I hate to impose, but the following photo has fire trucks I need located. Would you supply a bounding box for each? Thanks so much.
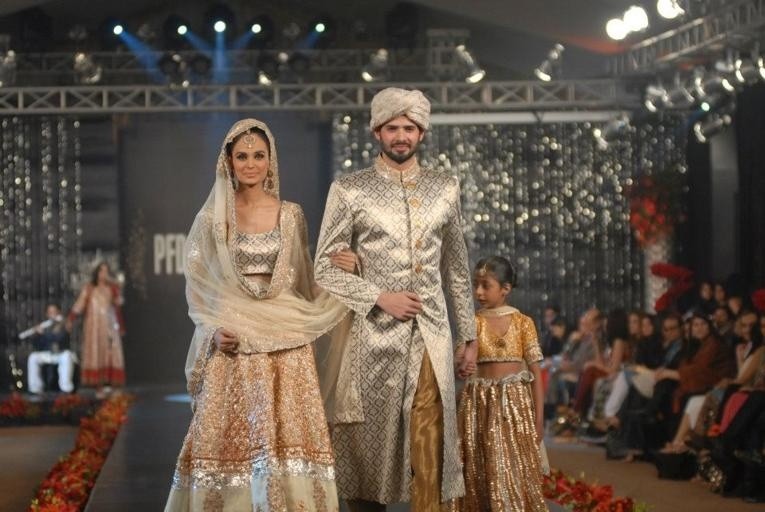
[18,315,62,339]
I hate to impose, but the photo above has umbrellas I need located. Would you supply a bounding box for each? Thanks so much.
[661,435,690,454]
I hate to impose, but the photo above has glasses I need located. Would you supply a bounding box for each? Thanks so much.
[534,42,567,82]
[455,43,486,87]
[694,110,733,144]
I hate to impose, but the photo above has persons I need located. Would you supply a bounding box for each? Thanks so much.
[67,261,127,390]
[544,278,765,469]
[456,257,551,512]
[314,88,479,512]
[24,302,75,395]
[165,119,356,512]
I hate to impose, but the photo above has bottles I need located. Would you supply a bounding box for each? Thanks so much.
[369,87,431,130]
